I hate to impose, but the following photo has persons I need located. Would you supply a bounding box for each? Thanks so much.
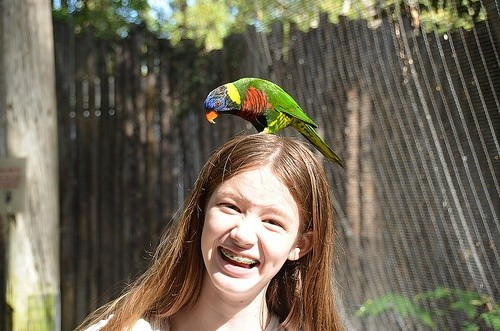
[74,132,345,331]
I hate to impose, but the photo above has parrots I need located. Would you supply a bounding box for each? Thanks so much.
[203,78,346,169]
